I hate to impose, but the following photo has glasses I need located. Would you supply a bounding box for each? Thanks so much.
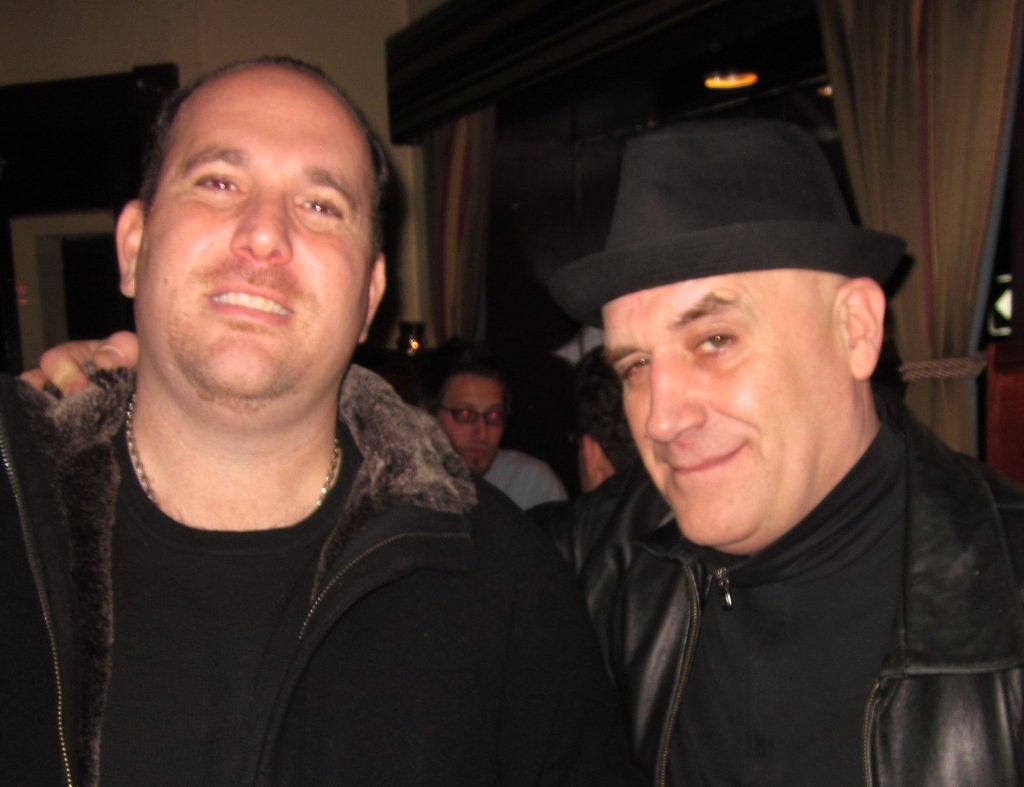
[438,403,506,426]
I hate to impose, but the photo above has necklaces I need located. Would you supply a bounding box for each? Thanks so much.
[125,386,340,523]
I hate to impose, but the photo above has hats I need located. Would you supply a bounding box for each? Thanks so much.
[548,120,912,330]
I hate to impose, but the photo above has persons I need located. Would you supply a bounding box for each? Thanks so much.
[570,343,639,495]
[0,54,642,787]
[416,334,570,511]
[18,116,1024,787]
